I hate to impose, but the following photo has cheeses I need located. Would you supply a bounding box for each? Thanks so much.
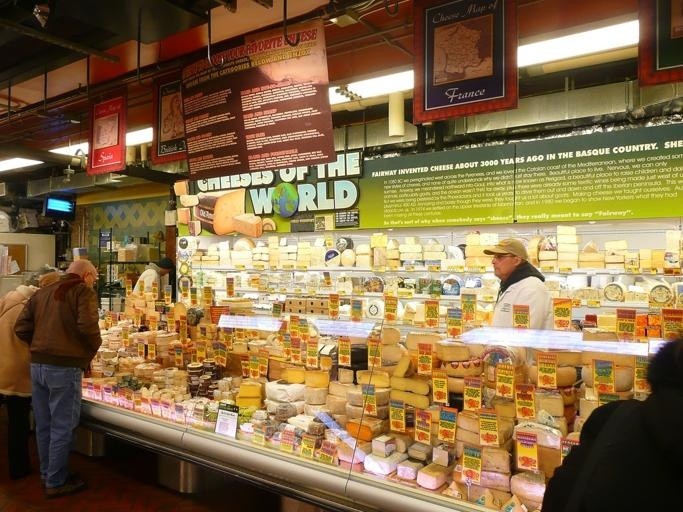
[81,224,683,512]
[173,180,276,237]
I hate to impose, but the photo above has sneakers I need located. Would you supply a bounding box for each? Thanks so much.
[44,478,87,497]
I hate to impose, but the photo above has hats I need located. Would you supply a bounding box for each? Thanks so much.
[484,239,528,261]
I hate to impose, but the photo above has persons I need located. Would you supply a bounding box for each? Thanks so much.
[14,258,103,499]
[0,270,62,481]
[483,239,554,329]
[133,258,174,300]
[540,331,683,512]
[164,95,184,138]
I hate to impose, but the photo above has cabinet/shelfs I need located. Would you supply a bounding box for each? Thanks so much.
[189,237,681,313]
[80,296,682,510]
